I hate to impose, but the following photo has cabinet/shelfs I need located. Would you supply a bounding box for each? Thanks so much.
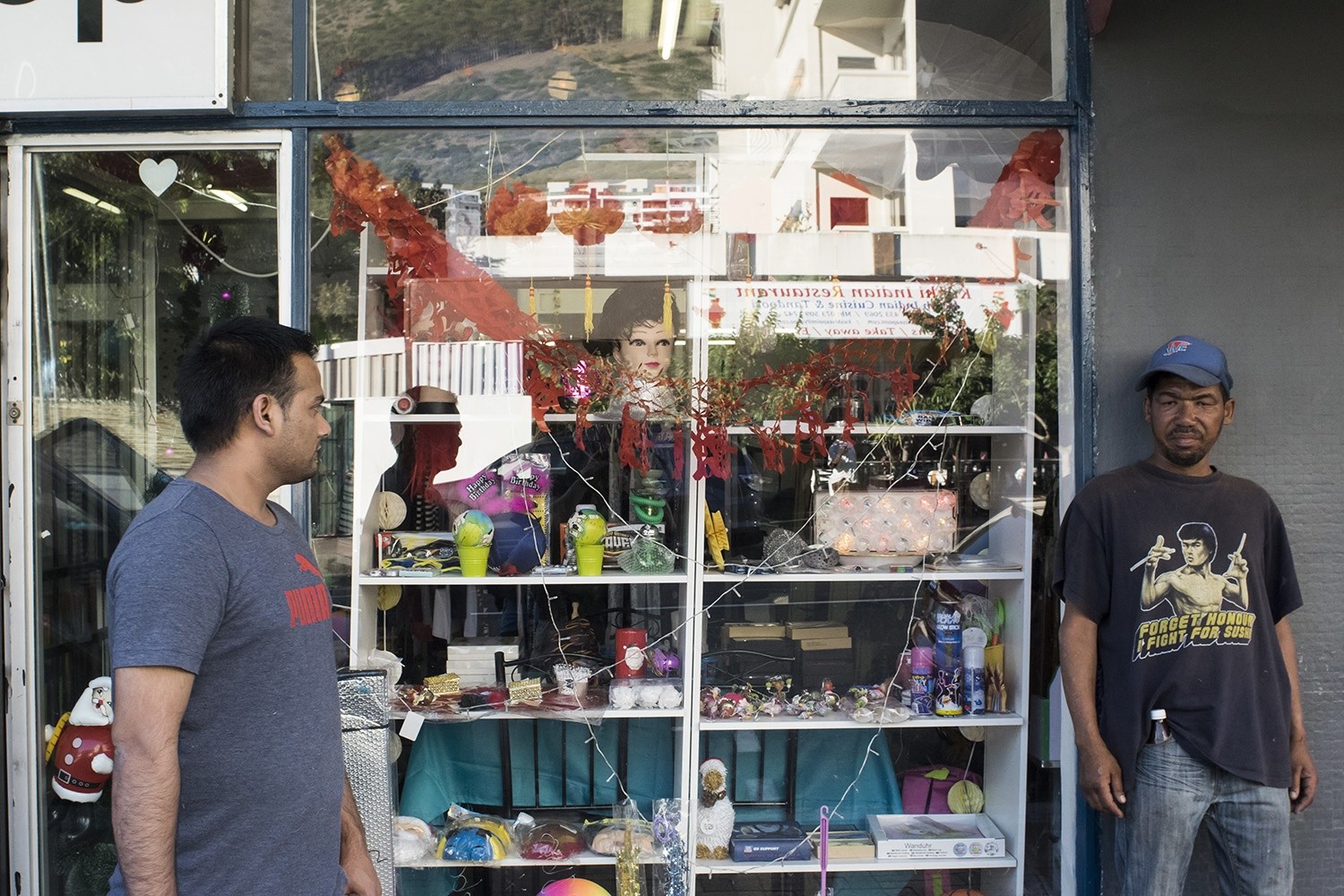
[698,272,1041,894]
[342,182,701,896]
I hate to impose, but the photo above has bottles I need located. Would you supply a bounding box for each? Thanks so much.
[1149,708,1169,745]
[965,645,986,714]
[910,647,933,714]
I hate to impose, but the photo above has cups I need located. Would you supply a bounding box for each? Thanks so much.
[574,545,604,577]
[614,626,648,678]
[456,548,489,577]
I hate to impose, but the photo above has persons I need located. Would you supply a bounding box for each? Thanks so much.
[586,282,682,418]
[1048,332,1320,895]
[827,430,860,487]
[103,316,383,896]
[367,384,467,691]
[45,676,113,849]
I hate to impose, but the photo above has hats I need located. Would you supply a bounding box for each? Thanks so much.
[1136,335,1233,398]
